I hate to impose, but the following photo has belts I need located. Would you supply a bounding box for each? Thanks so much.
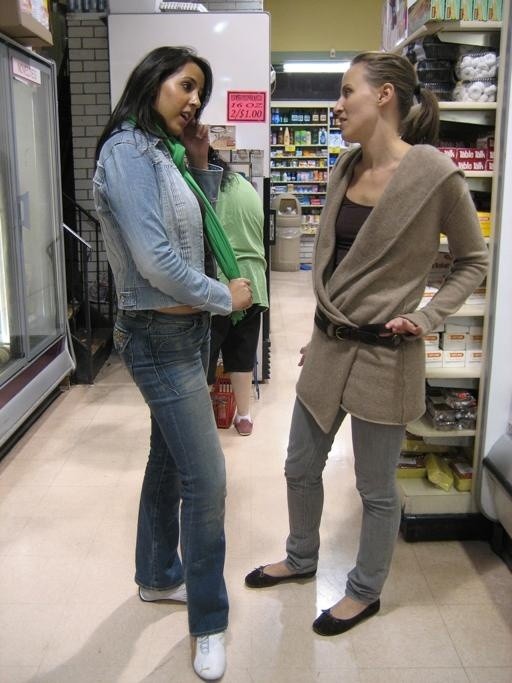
[314,305,404,348]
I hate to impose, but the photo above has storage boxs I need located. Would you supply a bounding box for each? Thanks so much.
[0,0,54,48]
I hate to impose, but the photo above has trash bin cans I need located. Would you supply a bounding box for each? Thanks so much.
[271,193,303,272]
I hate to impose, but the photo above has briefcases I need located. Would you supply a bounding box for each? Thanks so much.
[210,375,237,428]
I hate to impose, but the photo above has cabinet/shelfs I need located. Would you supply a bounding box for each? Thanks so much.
[330,106,361,177]
[382,21,504,545]
[268,106,329,253]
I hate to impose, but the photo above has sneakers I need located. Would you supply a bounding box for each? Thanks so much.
[193,630,226,680]
[234,414,253,435]
[139,580,188,602]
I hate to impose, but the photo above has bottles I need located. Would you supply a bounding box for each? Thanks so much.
[270,125,328,144]
[270,107,325,123]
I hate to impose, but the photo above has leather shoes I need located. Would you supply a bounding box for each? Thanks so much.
[245,563,316,587]
[313,597,380,635]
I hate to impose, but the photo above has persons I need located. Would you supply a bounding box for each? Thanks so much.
[207,146,270,437]
[92,45,255,683]
[247,52,490,634]
